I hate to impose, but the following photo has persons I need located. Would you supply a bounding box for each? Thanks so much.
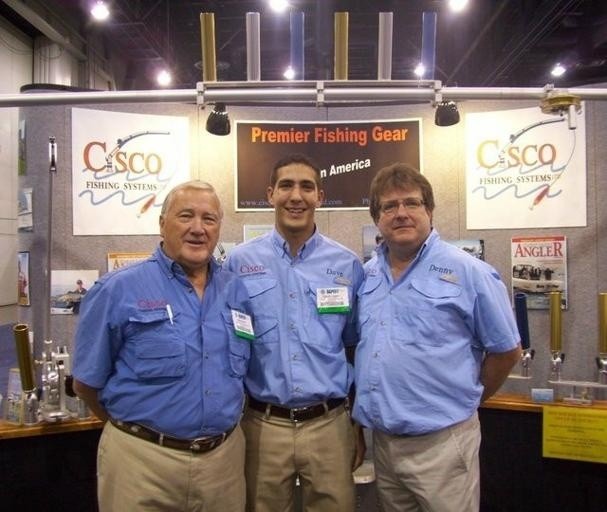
[73,280,87,295]
[351,162,523,511]
[228,155,368,510]
[71,180,252,511]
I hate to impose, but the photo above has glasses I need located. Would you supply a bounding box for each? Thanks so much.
[381,198,423,212]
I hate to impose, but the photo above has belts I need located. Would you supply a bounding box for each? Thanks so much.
[106,413,238,453]
[398,433,429,438]
[249,398,344,420]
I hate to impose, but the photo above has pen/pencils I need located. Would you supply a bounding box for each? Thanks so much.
[166,304,175,326]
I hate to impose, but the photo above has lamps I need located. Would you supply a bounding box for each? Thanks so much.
[197,78,232,136]
[431,80,462,126]
